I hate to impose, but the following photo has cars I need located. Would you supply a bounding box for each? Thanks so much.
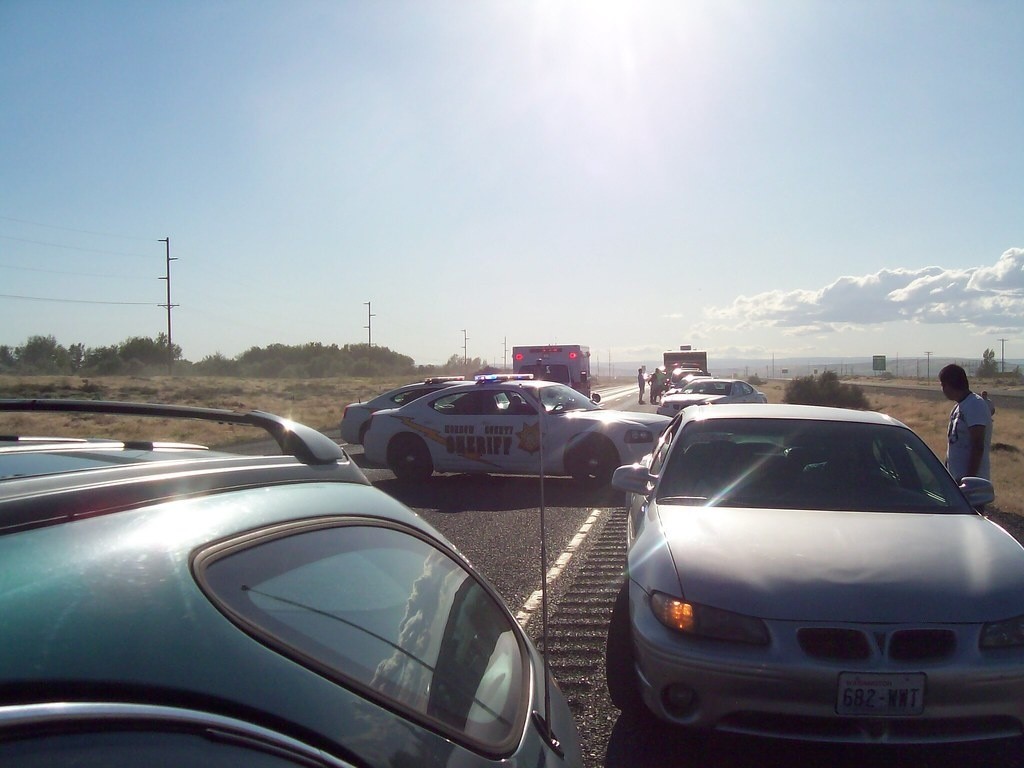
[661,376,722,400]
[361,377,671,490]
[658,379,768,416]
[343,377,566,447]
[605,404,1024,749]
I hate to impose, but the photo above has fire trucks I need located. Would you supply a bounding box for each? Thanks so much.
[512,345,591,397]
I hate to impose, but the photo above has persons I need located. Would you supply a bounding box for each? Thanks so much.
[638,368,646,404]
[647,368,670,404]
[507,396,529,411]
[938,364,995,514]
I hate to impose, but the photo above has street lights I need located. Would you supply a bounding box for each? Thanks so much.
[997,339,1008,372]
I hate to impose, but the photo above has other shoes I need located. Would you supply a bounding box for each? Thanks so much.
[639,400,645,405]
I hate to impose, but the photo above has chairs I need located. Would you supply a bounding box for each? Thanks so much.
[683,441,903,505]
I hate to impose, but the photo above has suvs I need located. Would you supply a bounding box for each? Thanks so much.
[666,366,705,390]
[1,396,579,768]
[663,345,711,383]
